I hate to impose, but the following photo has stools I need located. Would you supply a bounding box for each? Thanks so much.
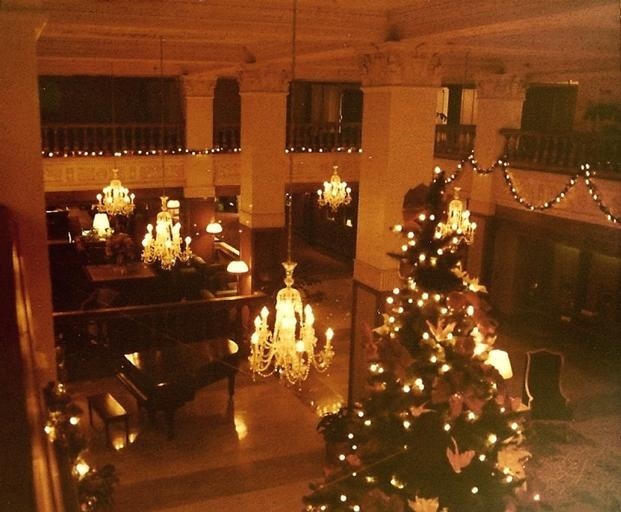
[87,391,129,447]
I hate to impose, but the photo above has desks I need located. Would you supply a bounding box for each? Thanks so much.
[86,261,226,305]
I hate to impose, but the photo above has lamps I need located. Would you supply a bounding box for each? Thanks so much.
[227,260,250,276]
[240,1,336,386]
[140,36,193,272]
[204,222,223,234]
[316,44,353,212]
[95,62,136,218]
[434,50,477,251]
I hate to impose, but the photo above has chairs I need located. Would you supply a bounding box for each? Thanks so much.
[522,350,571,445]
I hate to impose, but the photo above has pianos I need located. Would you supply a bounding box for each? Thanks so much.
[114,336,239,439]
